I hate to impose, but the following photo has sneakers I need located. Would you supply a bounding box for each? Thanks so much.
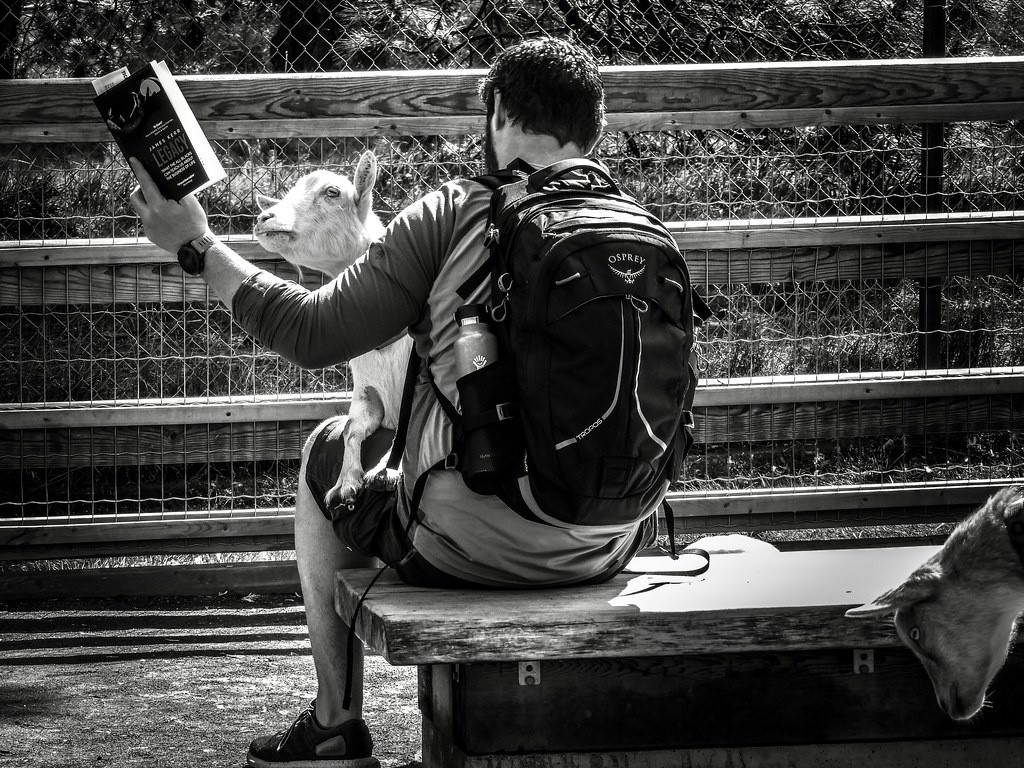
[246,698,380,768]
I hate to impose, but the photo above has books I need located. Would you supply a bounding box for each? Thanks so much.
[94,60,228,204]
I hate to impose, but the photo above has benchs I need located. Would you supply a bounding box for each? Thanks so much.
[332,545,945,768]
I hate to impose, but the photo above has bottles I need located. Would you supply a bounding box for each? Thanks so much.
[452,303,499,379]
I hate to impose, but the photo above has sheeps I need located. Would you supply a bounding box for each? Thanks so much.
[251,150,416,508]
[844,483,1024,722]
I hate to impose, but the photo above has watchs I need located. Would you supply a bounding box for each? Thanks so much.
[177,231,220,276]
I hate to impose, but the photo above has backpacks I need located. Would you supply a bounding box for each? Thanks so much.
[419,157,702,528]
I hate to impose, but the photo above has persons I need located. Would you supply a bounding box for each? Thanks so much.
[127,38,660,768]
